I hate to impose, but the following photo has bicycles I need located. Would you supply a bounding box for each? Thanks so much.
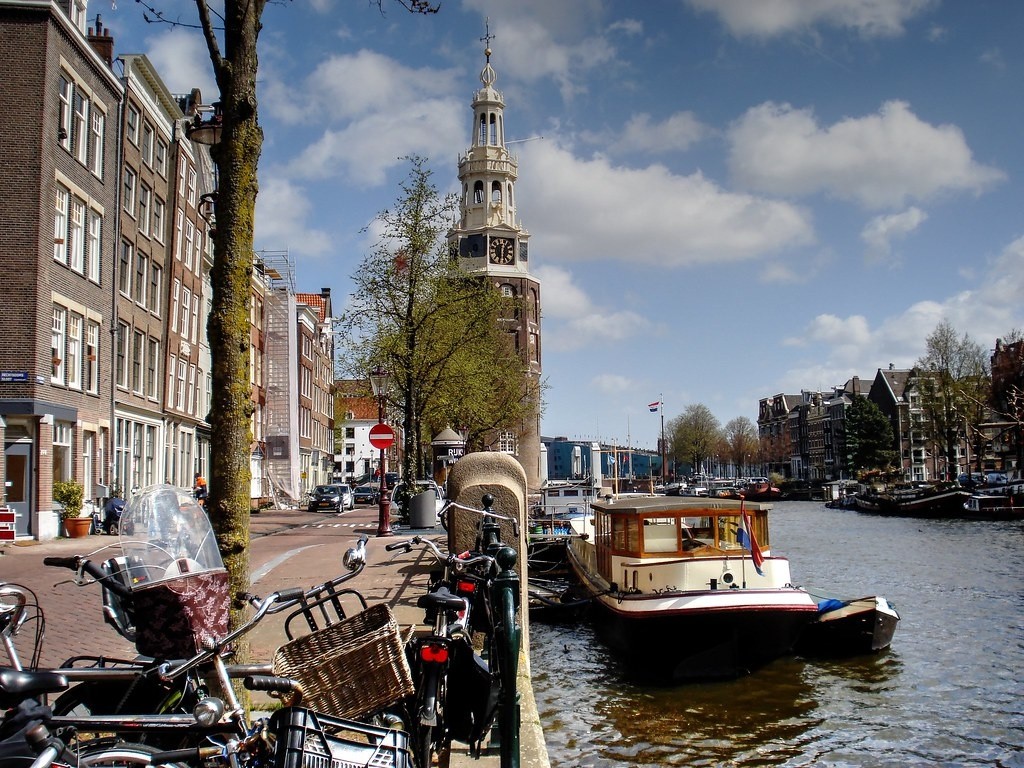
[0,484,520,768]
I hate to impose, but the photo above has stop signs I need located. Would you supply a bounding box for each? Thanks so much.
[369,424,394,449]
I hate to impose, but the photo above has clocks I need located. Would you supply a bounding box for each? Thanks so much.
[490,238,514,265]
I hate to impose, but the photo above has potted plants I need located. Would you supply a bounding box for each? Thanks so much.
[53,479,93,539]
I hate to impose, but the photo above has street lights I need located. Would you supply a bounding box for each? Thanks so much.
[370,450,374,469]
[368,362,396,537]
[349,449,354,484]
[743,453,751,473]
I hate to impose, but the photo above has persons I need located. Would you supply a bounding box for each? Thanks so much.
[193,473,207,509]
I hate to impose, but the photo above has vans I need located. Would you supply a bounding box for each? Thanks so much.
[747,477,775,486]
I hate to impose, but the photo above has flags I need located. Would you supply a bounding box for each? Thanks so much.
[623,455,628,462]
[736,502,766,577]
[608,455,616,467]
[648,401,660,413]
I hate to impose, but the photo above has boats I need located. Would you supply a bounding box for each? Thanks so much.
[565,496,818,646]
[653,480,745,499]
[739,482,783,500]
[963,495,1024,519]
[855,487,972,519]
[792,596,900,654]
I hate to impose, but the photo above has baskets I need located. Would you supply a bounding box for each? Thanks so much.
[271,603,415,726]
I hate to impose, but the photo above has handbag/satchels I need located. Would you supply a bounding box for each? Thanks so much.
[448,637,502,744]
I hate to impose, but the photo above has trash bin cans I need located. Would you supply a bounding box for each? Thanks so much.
[408,489,436,527]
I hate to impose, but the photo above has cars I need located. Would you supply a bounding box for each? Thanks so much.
[987,475,1008,484]
[353,486,380,506]
[1005,479,1024,491]
[331,484,355,511]
[387,480,446,529]
[958,475,981,485]
[351,474,376,490]
[373,477,381,483]
[308,485,344,513]
[386,472,400,485]
